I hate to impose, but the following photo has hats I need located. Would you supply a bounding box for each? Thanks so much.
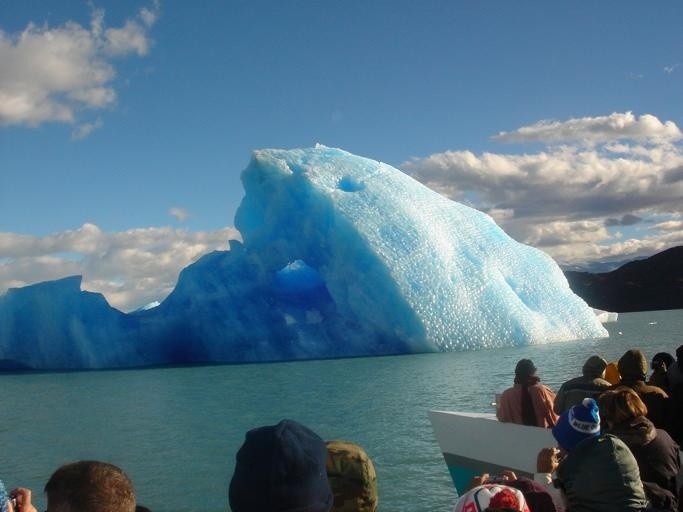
[453,478,557,512]
[552,397,601,453]
[229,419,378,512]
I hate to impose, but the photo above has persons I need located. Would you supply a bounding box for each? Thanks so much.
[228,418,335,511]
[4,459,136,512]
[454,345,681,510]
[325,440,378,511]
